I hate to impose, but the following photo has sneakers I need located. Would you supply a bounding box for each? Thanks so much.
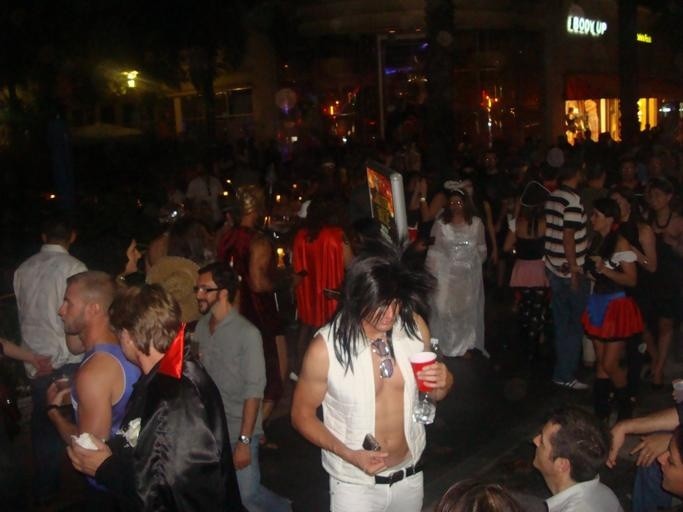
[549,359,595,390]
[288,367,300,382]
[640,362,667,394]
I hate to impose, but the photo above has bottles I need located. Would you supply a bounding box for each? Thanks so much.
[411,336,442,426]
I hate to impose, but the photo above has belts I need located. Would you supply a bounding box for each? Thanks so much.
[375,464,423,485]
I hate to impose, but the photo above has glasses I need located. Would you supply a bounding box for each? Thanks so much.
[371,338,393,379]
[192,286,221,294]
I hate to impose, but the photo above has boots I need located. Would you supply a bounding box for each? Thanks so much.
[594,376,637,424]
